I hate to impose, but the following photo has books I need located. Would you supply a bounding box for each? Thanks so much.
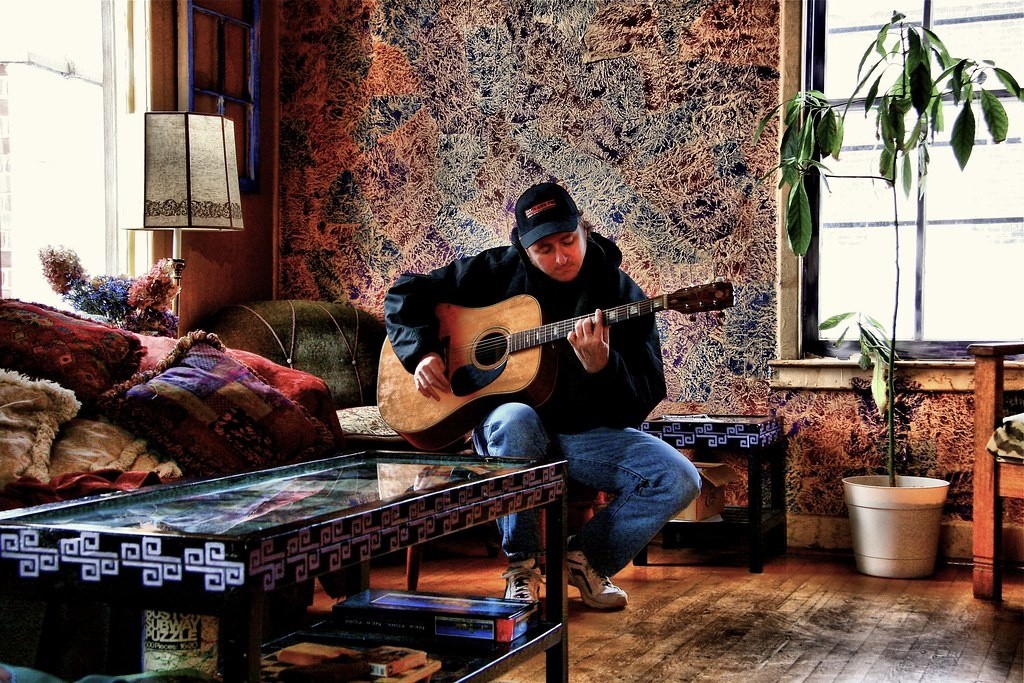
[279,642,442,683]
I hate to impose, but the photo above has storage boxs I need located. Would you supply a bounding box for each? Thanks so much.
[331,586,544,645]
[671,461,727,521]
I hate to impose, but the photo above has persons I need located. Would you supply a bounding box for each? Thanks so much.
[383,182,702,609]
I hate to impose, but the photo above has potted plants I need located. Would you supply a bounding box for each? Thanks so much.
[748,9,1024,581]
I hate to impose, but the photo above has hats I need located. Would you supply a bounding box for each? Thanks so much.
[515,182,579,249]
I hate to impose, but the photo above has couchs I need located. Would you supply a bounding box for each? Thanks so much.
[0,301,346,620]
[198,298,385,407]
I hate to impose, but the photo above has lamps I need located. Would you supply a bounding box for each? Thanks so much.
[115,107,246,326]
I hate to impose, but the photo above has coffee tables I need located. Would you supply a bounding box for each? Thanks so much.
[0,443,574,683]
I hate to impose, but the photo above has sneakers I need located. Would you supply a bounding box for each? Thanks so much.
[501,559,546,601]
[567,535,628,609]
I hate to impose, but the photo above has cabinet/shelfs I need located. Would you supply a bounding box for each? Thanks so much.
[633,411,786,573]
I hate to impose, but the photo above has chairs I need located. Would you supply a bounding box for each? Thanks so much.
[965,340,1024,615]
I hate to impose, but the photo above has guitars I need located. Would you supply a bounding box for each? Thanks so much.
[376,275,738,453]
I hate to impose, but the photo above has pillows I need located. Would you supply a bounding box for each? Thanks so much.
[0,296,148,409]
[0,363,82,486]
[93,330,341,483]
